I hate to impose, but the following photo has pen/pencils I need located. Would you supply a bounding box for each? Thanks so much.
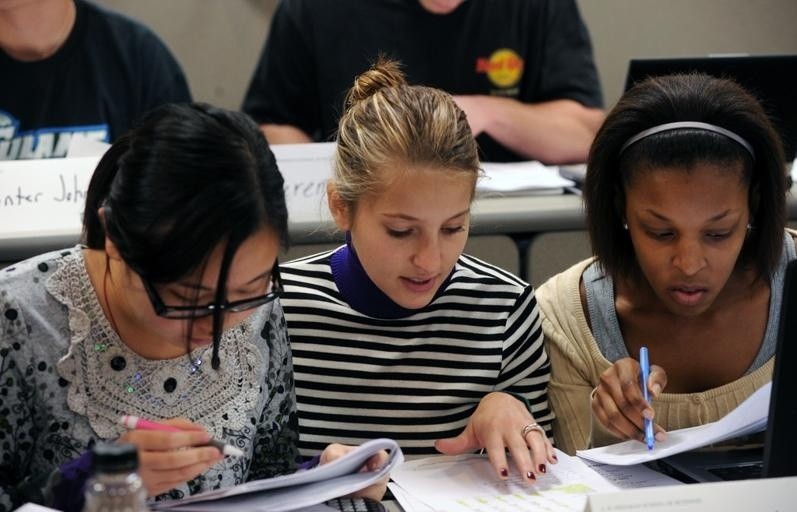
[120,413,251,461]
[639,346,654,452]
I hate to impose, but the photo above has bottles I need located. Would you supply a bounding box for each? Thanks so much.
[81,441,148,512]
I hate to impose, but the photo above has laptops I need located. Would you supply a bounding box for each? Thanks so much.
[559,55,796,186]
[644,261,797,485]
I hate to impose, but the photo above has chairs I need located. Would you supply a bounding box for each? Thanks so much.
[458,230,523,279]
[523,229,597,292]
[268,236,348,271]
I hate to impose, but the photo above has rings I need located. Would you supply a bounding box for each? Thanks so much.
[520,423,545,439]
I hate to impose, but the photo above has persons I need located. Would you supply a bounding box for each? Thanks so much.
[272,53,559,486]
[0,0,194,163]
[0,101,390,511]
[238,0,605,166]
[535,71,795,457]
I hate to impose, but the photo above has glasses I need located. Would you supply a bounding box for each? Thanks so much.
[140,255,284,320]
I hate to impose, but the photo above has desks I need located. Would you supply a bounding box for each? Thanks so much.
[0,152,591,245]
